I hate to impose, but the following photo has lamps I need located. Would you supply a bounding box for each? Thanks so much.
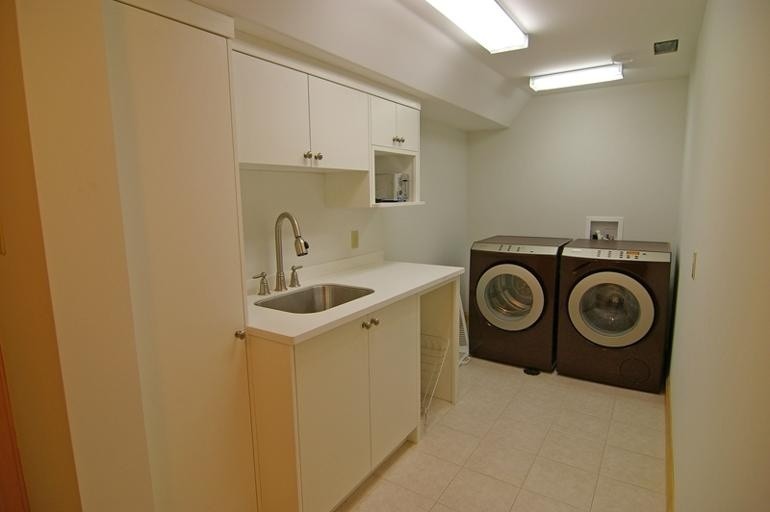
[528,62,624,93]
[427,0,529,55]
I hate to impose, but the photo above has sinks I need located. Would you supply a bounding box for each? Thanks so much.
[254,284,375,313]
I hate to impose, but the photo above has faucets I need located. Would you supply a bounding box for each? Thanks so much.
[274,211,308,292]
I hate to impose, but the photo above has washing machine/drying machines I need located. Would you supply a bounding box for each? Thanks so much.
[556,238,672,394]
[468,235,573,373]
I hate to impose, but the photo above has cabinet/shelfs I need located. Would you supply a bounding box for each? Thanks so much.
[230,41,368,171]
[0,1,263,510]
[247,296,421,511]
[369,90,425,210]
